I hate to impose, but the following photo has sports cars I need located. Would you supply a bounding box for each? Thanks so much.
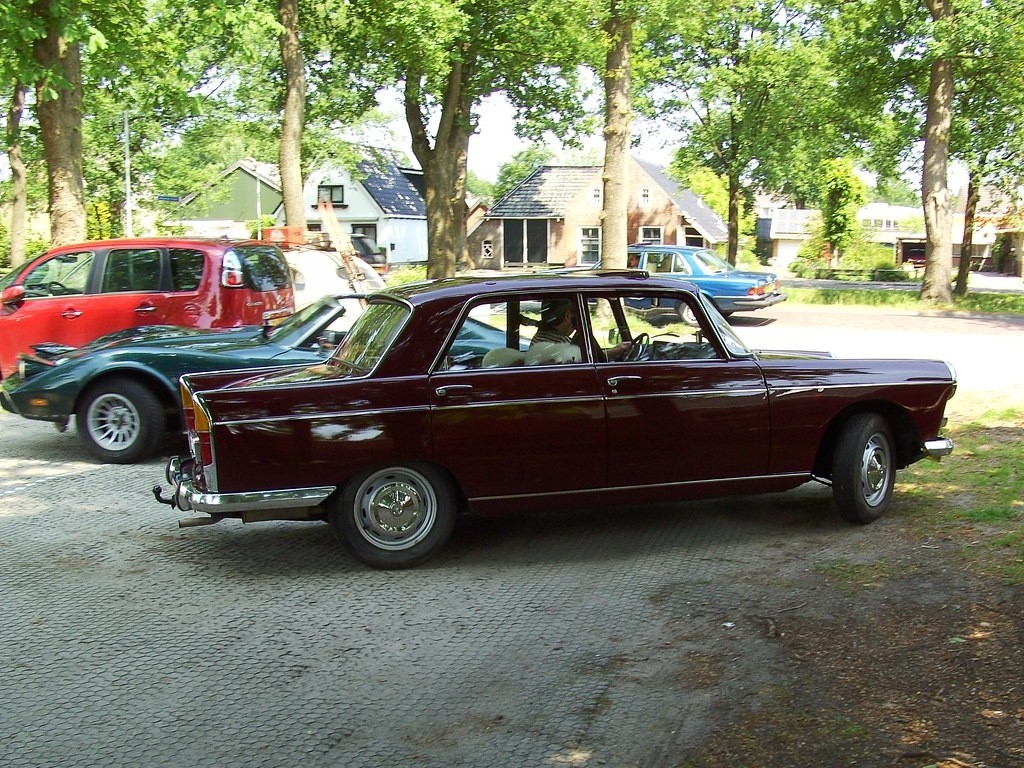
[1,293,534,464]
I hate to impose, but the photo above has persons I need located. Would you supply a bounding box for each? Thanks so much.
[529,296,631,362]
[629,254,638,268]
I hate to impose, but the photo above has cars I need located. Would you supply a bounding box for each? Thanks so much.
[279,247,391,335]
[0,236,295,384]
[153,263,958,571]
[590,241,788,322]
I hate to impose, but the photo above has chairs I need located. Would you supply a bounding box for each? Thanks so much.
[524,342,582,366]
[483,347,524,368]
